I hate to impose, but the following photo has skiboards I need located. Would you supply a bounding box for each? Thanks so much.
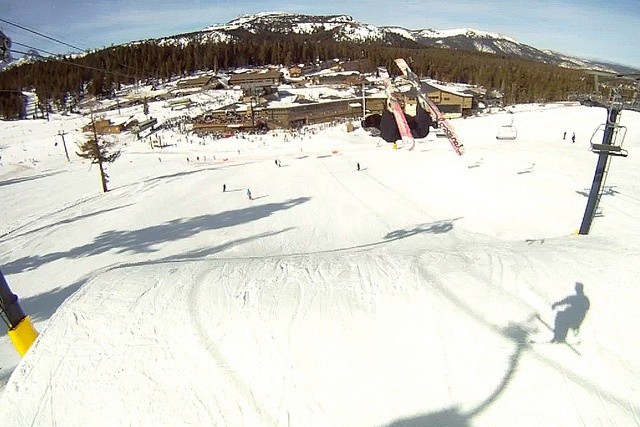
[377,59,465,156]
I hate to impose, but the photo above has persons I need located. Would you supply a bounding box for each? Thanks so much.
[361,90,438,143]
